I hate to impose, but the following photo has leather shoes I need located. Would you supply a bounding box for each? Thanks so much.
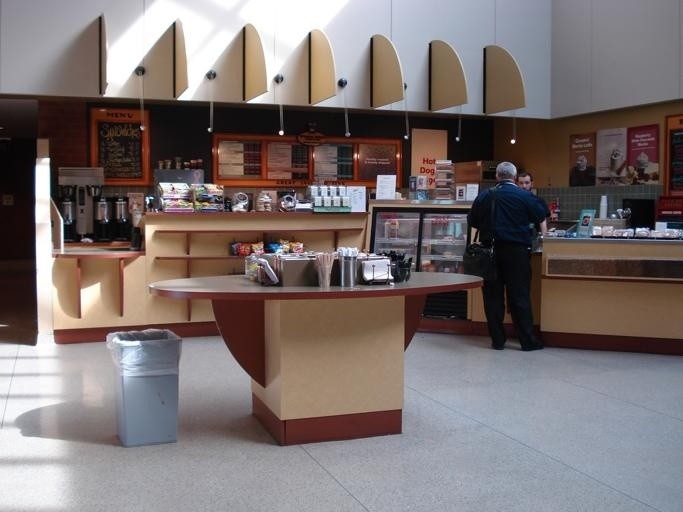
[520,340,544,351]
[491,339,504,350]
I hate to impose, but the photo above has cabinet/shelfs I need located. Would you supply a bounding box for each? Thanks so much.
[53,213,371,346]
[368,198,490,336]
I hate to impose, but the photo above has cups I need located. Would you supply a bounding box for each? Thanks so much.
[609,158,623,171]
[576,162,587,170]
[317,264,331,288]
[391,267,410,283]
[637,168,644,178]
[445,222,463,238]
[338,255,357,286]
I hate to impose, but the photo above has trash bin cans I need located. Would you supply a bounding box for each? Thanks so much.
[107,329,182,446]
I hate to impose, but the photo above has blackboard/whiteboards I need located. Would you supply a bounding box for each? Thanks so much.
[662,114,682,195]
[91,108,151,186]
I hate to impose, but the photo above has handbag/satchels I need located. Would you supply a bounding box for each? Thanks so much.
[463,243,497,280]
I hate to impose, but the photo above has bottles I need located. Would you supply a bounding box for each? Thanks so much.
[609,208,631,219]
[599,195,607,219]
[548,197,560,222]
[231,191,296,213]
[130,201,142,250]
[155,157,202,170]
[384,217,399,239]
[410,261,464,273]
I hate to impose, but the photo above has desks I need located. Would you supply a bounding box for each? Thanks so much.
[148,271,484,447]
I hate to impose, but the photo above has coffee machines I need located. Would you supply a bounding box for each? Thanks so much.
[55,168,128,243]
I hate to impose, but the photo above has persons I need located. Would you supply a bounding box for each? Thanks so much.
[466,162,548,354]
[516,172,533,192]
[568,154,594,187]
